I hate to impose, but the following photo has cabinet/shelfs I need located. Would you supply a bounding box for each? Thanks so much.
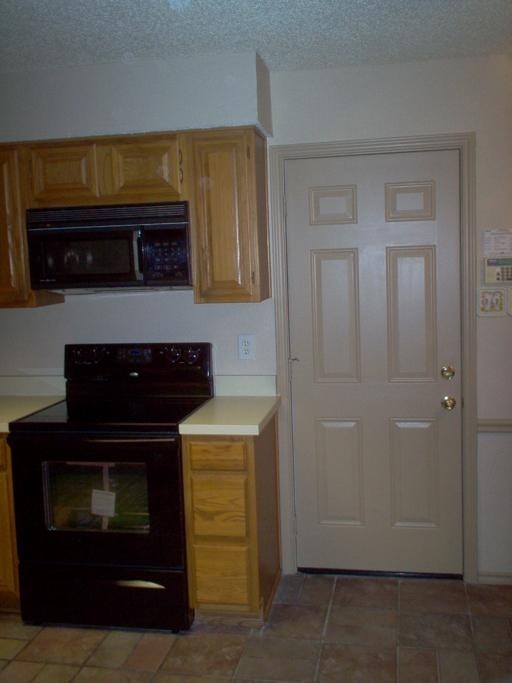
[0,124,270,309]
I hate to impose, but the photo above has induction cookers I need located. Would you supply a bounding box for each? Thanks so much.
[9,395,211,432]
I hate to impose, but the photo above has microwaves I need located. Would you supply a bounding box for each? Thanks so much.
[26,201,193,290]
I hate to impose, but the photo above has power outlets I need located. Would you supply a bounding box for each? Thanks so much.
[238,334,257,361]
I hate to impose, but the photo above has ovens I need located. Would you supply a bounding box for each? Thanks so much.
[6,434,197,633]
